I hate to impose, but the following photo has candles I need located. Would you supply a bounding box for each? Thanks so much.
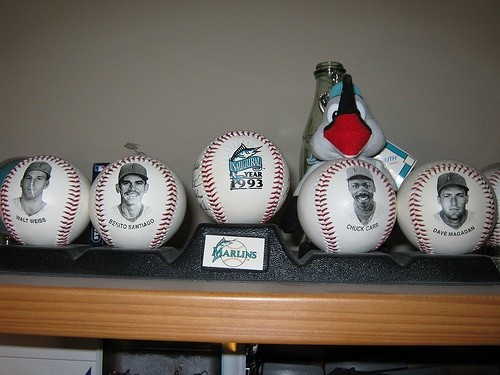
[220,343,247,375]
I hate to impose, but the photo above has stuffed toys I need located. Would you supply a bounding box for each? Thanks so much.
[310,74,386,163]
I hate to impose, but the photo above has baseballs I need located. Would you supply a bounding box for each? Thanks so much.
[0,156,93,246]
[296,159,397,256]
[396,159,499,257]
[190,129,291,223]
[87,155,188,252]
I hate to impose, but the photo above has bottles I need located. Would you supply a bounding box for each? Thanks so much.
[298,59,347,182]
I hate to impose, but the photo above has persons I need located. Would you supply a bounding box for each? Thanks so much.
[346,165,381,226]
[13,161,56,220]
[112,163,155,223]
[435,172,476,232]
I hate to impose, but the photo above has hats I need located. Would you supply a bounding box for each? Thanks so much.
[347,166,374,182]
[23,161,51,178]
[436,172,469,196]
[117,163,148,181]
